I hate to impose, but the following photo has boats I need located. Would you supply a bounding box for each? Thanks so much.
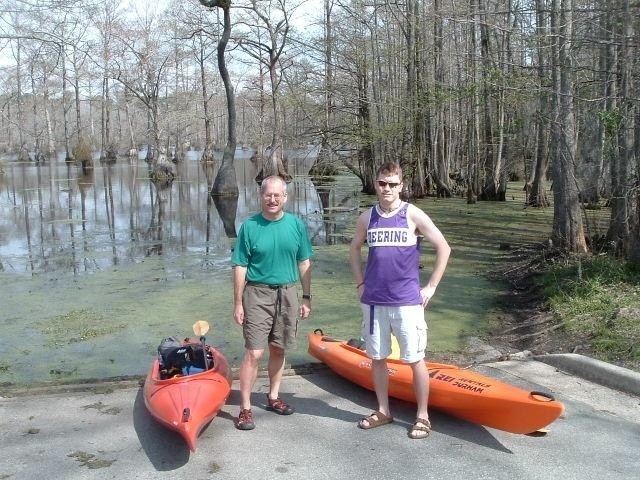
[306,328,564,439]
[141,334,229,453]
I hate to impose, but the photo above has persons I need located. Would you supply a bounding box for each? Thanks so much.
[231,176,313,430]
[350,162,452,438]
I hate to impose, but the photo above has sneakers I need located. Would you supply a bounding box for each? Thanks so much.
[266,393,295,415]
[237,409,255,430]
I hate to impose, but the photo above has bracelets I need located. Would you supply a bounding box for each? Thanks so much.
[355,280,365,289]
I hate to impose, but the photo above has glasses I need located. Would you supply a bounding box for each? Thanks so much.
[379,181,399,188]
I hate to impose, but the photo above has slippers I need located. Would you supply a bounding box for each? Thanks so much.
[410,418,432,439]
[359,412,393,429]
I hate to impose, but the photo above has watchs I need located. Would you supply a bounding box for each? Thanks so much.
[304,292,314,301]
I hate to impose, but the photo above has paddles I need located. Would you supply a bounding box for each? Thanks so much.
[193,321,209,370]
